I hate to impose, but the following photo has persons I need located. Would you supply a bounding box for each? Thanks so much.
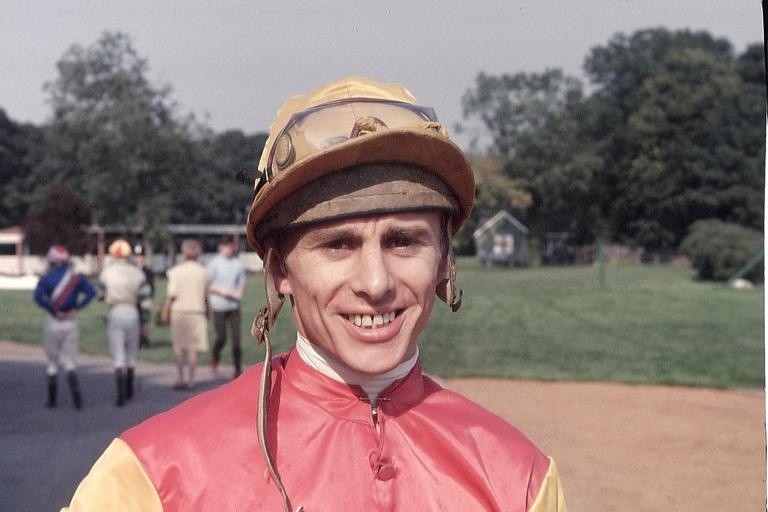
[97,238,154,407]
[160,238,212,391]
[58,76,570,512]
[131,242,154,350]
[206,236,247,380]
[32,244,97,410]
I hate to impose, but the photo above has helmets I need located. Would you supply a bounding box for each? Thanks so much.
[246,78,476,258]
[47,246,69,263]
[110,239,133,258]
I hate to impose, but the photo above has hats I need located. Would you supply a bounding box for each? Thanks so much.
[255,161,459,239]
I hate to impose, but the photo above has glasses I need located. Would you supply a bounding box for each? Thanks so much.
[253,98,438,197]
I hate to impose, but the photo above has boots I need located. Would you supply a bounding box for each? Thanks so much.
[45,369,134,410]
